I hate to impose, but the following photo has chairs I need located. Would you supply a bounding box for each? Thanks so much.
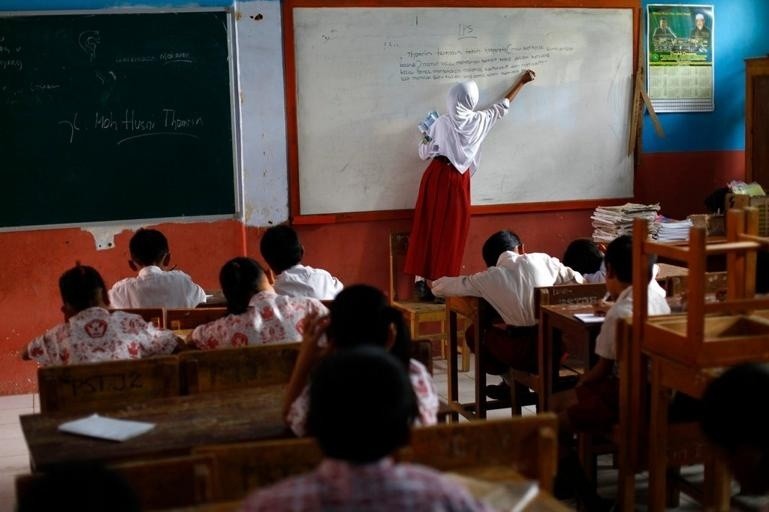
[394,412,559,498]
[179,342,305,391]
[37,355,180,414]
[195,433,324,497]
[625,208,769,473]
[389,230,471,373]
[13,454,215,512]
[533,283,616,410]
[109,307,164,332]
[164,308,230,330]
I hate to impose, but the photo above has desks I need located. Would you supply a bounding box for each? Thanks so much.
[647,351,743,511]
[17,386,294,471]
[442,294,521,423]
[539,303,607,477]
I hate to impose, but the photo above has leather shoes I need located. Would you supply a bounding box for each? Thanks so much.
[416,280,428,300]
[487,378,511,401]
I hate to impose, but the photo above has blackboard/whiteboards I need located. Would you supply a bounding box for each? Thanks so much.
[0,7,244,233]
[279,1,642,226]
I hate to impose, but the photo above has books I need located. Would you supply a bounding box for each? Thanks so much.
[56,412,156,443]
[417,110,440,144]
[590,202,694,243]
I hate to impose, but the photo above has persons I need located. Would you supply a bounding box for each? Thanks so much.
[689,14,711,47]
[279,283,441,439]
[546,233,677,438]
[21,265,186,370]
[184,256,330,350]
[561,237,660,284]
[259,224,344,301]
[108,228,207,317]
[429,229,586,401]
[652,18,677,42]
[403,68,536,302]
[238,342,477,512]
